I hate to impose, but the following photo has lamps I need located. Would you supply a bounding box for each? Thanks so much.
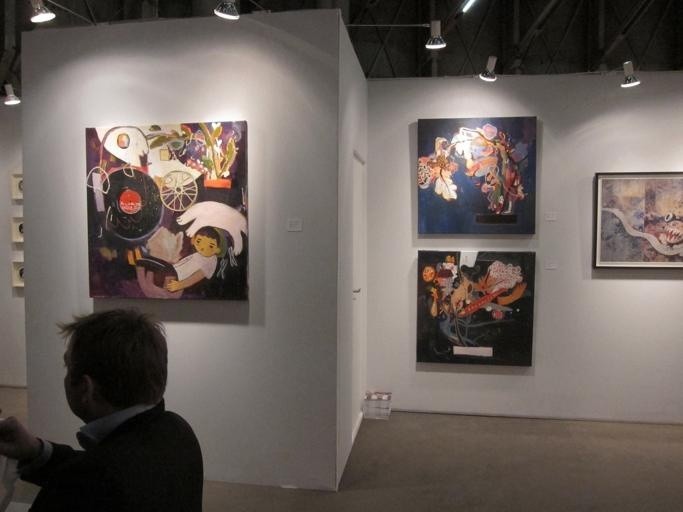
[479,56,498,83]
[214,0,264,21]
[345,20,447,50]
[620,61,641,89]
[4,83,21,105]
[30,0,95,25]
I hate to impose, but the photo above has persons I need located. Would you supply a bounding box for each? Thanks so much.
[0,305,204,512]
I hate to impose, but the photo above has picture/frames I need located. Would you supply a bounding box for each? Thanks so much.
[593,172,683,268]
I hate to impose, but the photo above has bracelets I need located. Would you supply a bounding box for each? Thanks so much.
[17,438,44,468]
